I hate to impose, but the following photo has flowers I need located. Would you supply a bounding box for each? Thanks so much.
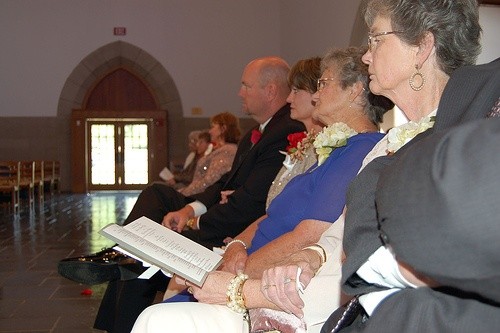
[311,118,358,169]
[286,127,314,162]
[385,110,439,156]
[250,129,260,146]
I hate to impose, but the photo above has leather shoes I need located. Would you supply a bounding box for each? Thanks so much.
[58,247,142,286]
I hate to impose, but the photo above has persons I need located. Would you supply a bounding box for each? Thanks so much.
[320,57,500,333]
[131,0,483,333]
[163,57,326,301]
[122,112,241,225]
[169,132,215,190]
[56,55,309,333]
[163,47,394,302]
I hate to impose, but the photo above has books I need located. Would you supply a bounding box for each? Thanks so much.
[98,216,226,288]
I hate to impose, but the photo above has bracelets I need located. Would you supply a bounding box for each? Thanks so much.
[225,240,246,251]
[226,274,249,313]
[187,218,194,226]
[302,246,325,262]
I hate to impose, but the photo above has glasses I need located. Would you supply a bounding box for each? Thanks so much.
[316,78,336,90]
[368,30,406,52]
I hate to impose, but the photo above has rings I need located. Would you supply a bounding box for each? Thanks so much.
[184,280,188,286]
[287,279,296,282]
[187,287,192,293]
[264,285,275,289]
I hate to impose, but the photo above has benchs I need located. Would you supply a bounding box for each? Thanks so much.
[21,160,36,207]
[44,159,56,197]
[0,160,21,213]
[55,160,62,196]
[35,160,44,202]
